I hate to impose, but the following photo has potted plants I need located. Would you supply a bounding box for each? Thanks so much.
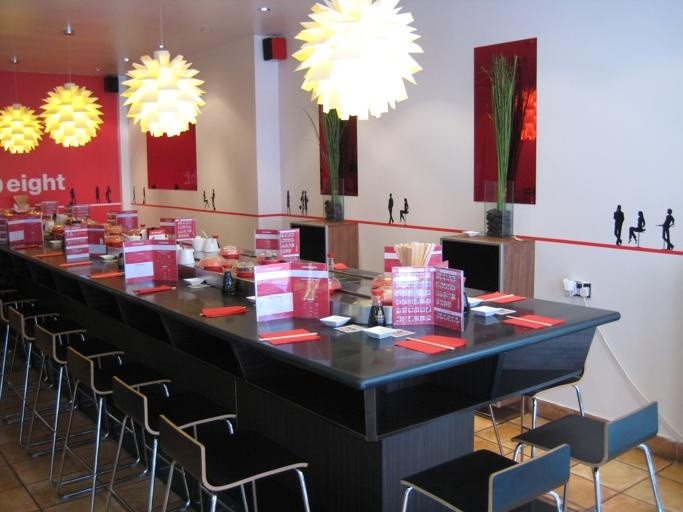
[302,106,350,223]
[478,54,520,239]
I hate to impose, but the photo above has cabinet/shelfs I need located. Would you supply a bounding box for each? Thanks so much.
[436,233,536,409]
[289,220,358,269]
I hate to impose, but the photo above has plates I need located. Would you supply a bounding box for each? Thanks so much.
[319,314,351,327]
[99,254,115,261]
[361,325,396,340]
[183,277,204,287]
[48,240,62,246]
[462,230,479,238]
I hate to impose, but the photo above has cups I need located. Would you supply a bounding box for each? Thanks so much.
[179,249,195,267]
[192,238,218,260]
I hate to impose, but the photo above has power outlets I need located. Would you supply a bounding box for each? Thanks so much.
[571,279,591,298]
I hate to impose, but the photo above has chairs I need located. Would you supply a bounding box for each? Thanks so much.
[26,322,141,487]
[104,376,191,512]
[55,346,148,500]
[157,414,310,512]
[2,306,98,448]
[510,401,670,512]
[400,443,570,511]
[0,300,55,399]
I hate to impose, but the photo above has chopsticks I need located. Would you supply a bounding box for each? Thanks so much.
[58,260,93,268]
[90,271,124,281]
[482,294,514,302]
[504,314,552,327]
[256,332,318,342]
[394,241,435,268]
[33,251,62,257]
[405,336,455,351]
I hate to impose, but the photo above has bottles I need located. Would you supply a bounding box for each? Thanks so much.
[367,288,385,327]
[220,263,234,296]
[324,253,335,281]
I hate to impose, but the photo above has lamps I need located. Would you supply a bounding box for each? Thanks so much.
[38,26,104,148]
[0,54,45,154]
[289,0,423,122]
[119,1,206,138]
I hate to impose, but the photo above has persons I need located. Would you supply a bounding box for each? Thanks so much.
[300,191,304,216]
[95,186,100,204]
[613,205,624,246]
[399,198,409,224]
[662,208,675,251]
[303,190,308,216]
[131,185,136,204]
[105,185,111,203]
[142,187,145,205]
[628,211,645,244]
[387,193,394,224]
[68,187,75,206]
[286,190,291,216]
[211,189,215,211]
[202,190,210,210]
